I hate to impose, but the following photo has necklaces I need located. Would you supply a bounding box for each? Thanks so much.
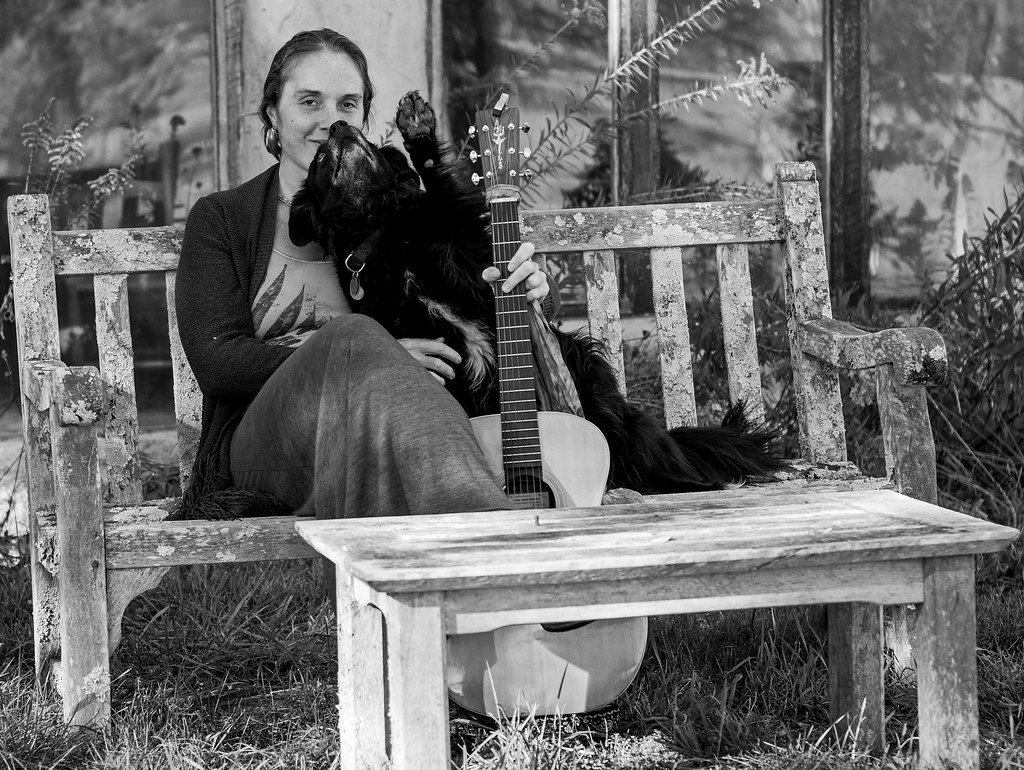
[277,192,291,206]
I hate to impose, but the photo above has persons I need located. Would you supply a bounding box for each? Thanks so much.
[174,28,550,516]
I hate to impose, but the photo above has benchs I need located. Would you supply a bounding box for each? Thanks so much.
[6,159,949,732]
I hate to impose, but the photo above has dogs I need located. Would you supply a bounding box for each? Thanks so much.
[289,92,799,497]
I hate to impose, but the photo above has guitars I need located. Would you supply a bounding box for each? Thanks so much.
[443,91,649,722]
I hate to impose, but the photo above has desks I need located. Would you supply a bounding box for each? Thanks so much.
[292,482,1019,770]
